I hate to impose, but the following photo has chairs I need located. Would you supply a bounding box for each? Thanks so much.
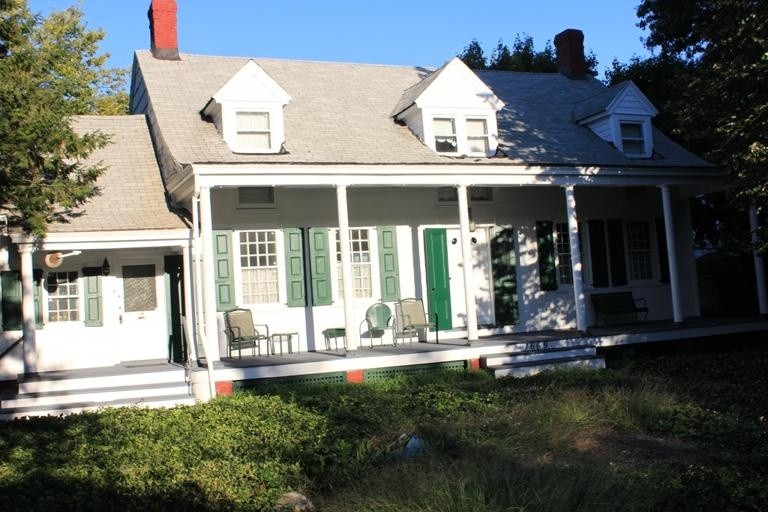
[398,297,439,345]
[366,303,396,349]
[226,308,270,360]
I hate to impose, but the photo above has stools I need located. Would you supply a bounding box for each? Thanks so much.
[273,333,300,357]
[323,328,346,351]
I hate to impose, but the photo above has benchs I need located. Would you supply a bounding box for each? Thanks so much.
[590,292,651,325]
